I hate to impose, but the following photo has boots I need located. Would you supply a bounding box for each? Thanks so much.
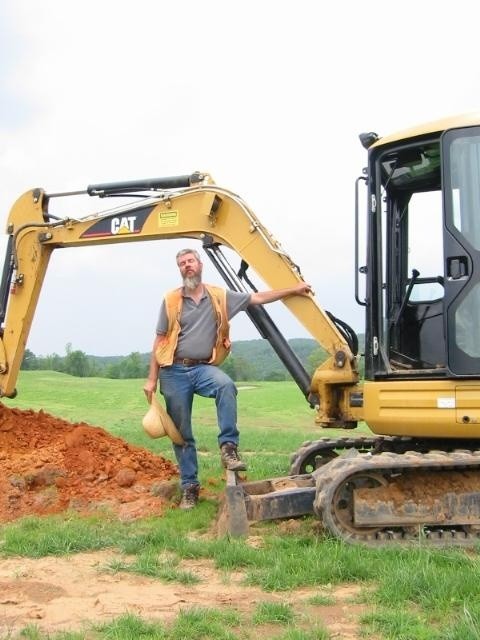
[220,441,248,472]
[177,482,199,510]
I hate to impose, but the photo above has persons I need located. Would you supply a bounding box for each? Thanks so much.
[143,248,316,511]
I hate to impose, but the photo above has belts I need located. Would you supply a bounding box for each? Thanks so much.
[171,357,209,366]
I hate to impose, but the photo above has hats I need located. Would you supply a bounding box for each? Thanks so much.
[143,393,186,446]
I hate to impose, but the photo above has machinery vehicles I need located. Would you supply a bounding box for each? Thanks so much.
[1,116,480,549]
